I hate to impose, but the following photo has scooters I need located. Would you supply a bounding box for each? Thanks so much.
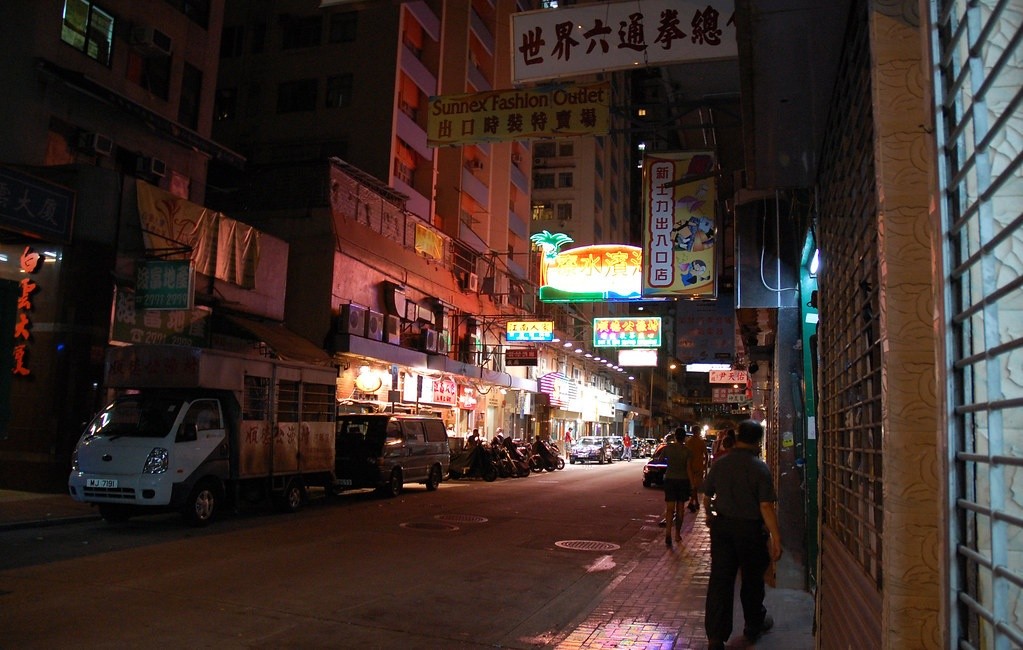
[449,434,565,482]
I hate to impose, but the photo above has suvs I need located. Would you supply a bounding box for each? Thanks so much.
[663,433,693,442]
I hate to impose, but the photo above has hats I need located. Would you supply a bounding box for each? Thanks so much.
[739,420,763,439]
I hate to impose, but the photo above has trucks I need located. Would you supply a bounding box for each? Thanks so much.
[69,344,338,527]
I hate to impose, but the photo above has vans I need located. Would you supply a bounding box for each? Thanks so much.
[335,414,450,497]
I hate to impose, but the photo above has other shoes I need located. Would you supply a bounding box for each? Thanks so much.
[666,536,672,544]
[675,537,681,540]
[695,503,701,510]
[744,615,773,637]
[708,639,724,649]
[687,503,697,513]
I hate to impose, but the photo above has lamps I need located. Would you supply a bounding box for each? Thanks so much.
[810,248,819,278]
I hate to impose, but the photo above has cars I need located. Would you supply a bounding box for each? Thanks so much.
[642,444,709,487]
[569,436,659,465]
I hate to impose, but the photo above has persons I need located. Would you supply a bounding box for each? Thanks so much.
[620,432,632,462]
[464,429,481,449]
[496,428,505,441]
[658,426,709,546]
[565,428,574,459]
[710,428,736,467]
[701,419,782,650]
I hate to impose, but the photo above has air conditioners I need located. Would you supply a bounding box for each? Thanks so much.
[134,28,173,60]
[383,314,401,347]
[337,303,365,338]
[463,272,478,294]
[364,309,384,342]
[142,156,167,179]
[532,157,545,169]
[416,328,448,356]
[511,154,523,163]
[734,395,745,403]
[78,131,113,160]
[472,159,485,171]
